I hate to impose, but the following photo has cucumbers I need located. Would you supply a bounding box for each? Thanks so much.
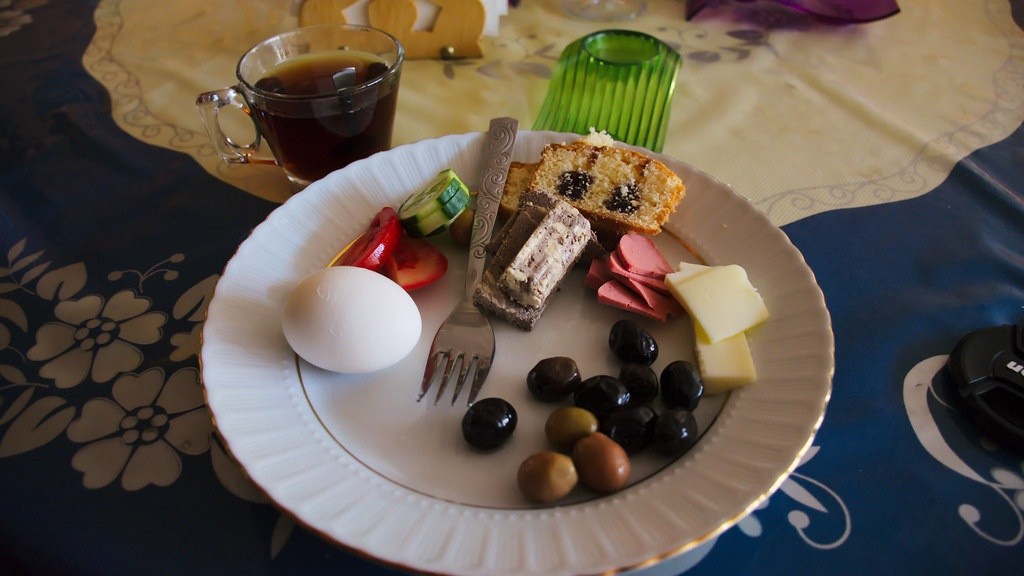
[394,166,473,239]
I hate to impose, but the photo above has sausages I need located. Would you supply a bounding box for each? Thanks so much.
[577,226,676,324]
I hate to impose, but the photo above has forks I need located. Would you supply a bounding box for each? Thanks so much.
[417,115,520,405]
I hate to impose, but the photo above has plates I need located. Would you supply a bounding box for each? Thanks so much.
[195,129,837,575]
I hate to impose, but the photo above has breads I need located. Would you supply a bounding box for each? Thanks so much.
[490,133,686,241]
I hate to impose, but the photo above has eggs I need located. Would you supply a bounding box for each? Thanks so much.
[280,265,431,380]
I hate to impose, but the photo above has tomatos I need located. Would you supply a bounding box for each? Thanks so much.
[333,203,447,291]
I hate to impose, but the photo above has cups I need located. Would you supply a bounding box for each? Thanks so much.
[529,28,682,154]
[196,24,406,194]
